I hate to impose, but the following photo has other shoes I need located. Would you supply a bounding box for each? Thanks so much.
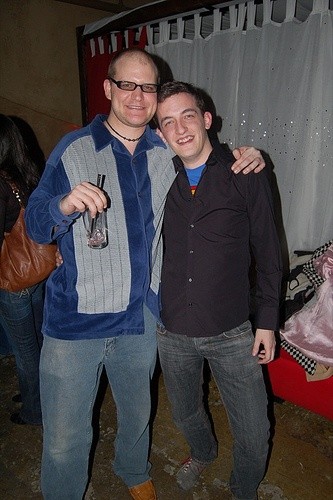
[13,393,21,402]
[175,456,210,491]
[231,469,257,500]
[10,413,27,425]
[128,480,156,500]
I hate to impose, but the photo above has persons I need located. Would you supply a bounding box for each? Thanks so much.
[150,81,286,500]
[0,114,63,425]
[22,47,268,500]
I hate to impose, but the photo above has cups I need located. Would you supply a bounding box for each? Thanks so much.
[83,207,109,249]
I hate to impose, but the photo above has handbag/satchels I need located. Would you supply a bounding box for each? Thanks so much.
[0,170,68,293]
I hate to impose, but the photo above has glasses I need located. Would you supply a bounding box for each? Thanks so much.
[109,76,159,93]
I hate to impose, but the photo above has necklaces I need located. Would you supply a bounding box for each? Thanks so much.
[106,120,146,142]
